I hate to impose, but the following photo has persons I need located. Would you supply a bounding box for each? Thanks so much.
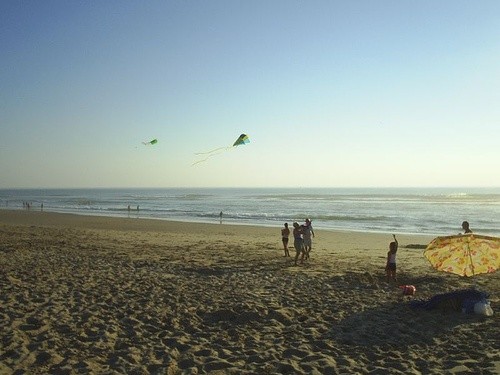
[385,234,398,282]
[303,218,315,259]
[292,222,304,266]
[281,223,290,257]
[462,221,472,234]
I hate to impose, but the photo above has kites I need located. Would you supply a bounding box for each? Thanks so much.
[192,134,251,167]
[140,138,158,145]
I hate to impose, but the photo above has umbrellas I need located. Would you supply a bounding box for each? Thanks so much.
[423,233,500,284]
